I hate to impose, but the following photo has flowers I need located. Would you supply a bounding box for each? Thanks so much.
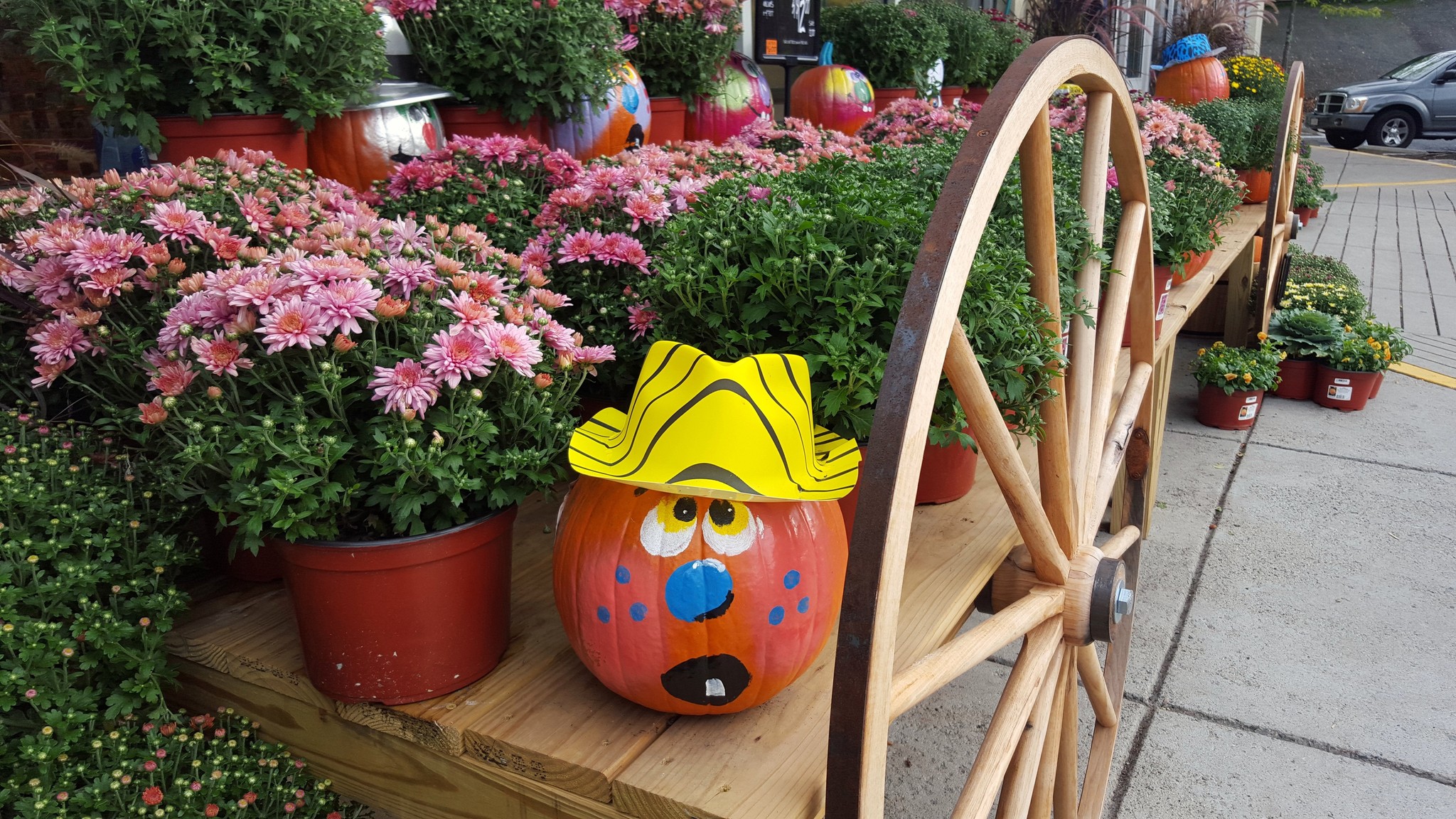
[1,0,1415,818]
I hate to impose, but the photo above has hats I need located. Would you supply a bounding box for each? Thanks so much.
[1150,33,1227,72]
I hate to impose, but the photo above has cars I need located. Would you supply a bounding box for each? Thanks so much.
[1306,49,1456,150]
